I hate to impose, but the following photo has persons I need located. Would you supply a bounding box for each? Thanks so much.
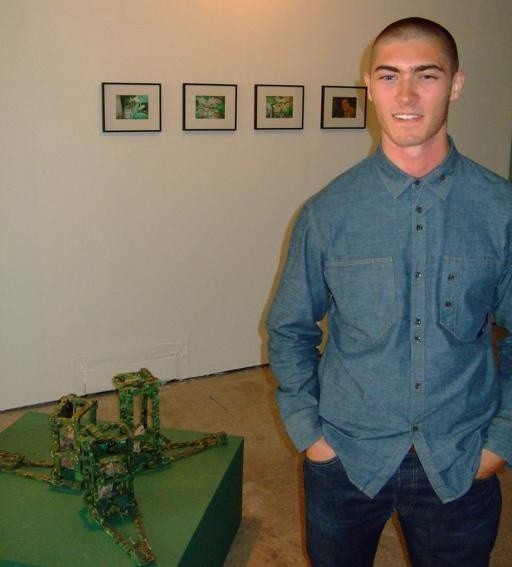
[265,15,512,567]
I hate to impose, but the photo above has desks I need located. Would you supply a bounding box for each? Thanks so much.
[0,410,245,567]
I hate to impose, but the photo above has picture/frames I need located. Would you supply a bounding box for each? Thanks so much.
[182,83,237,130]
[101,82,161,132]
[254,84,304,130]
[320,86,367,128]
